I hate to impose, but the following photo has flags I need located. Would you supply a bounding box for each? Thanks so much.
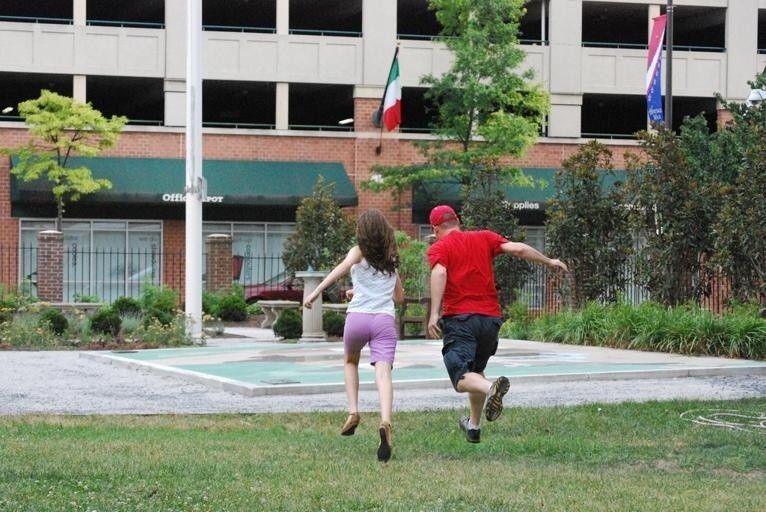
[383,56,402,133]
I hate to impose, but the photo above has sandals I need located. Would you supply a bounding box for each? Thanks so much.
[377,422,392,462]
[340,413,360,435]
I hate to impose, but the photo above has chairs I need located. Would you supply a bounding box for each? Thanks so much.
[397,297,432,340]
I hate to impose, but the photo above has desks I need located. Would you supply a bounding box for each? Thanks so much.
[257,299,301,330]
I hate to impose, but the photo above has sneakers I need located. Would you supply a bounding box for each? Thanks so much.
[459,416,481,443]
[483,376,510,422]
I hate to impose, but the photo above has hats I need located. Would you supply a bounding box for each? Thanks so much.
[430,205,457,230]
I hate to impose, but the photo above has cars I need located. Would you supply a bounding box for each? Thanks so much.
[244,263,329,305]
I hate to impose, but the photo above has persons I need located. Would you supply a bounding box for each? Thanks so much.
[426,205,569,442]
[304,208,405,463]
